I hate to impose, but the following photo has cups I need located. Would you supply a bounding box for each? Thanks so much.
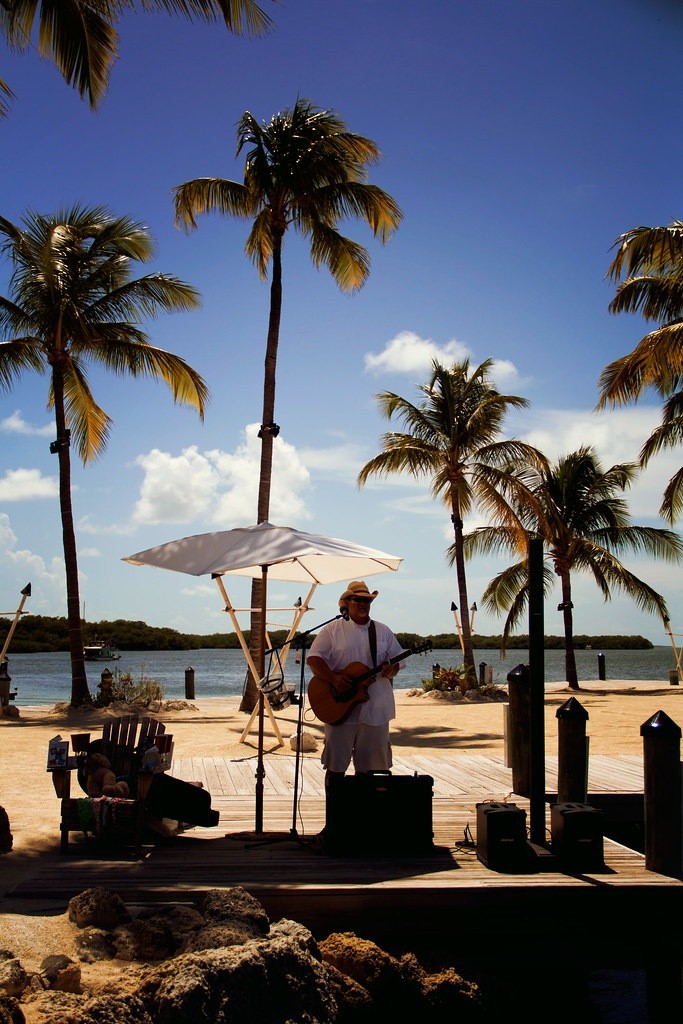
[71,733,82,752]
[154,734,168,754]
[81,733,90,750]
[166,734,174,752]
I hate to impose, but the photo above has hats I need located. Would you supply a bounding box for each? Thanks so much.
[338,579,378,609]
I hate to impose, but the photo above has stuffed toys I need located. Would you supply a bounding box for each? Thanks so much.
[81,752,129,799]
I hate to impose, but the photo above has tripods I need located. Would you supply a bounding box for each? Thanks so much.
[245,618,339,853]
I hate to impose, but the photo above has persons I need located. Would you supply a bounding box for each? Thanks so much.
[305,578,406,847]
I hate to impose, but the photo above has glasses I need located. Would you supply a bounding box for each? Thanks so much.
[344,598,373,604]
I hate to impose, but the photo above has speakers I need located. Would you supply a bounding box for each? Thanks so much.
[477,801,525,869]
[551,801,606,872]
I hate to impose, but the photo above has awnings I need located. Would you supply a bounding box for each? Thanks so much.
[119,519,403,747]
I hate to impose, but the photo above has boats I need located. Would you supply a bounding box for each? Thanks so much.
[82,634,121,662]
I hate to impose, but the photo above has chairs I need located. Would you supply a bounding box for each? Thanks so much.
[45,714,174,863]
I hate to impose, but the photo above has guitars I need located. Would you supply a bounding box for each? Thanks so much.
[307,638,433,728]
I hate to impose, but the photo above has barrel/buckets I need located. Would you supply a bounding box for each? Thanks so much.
[257,673,291,711]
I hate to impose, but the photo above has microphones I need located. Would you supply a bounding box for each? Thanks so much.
[340,605,349,621]
